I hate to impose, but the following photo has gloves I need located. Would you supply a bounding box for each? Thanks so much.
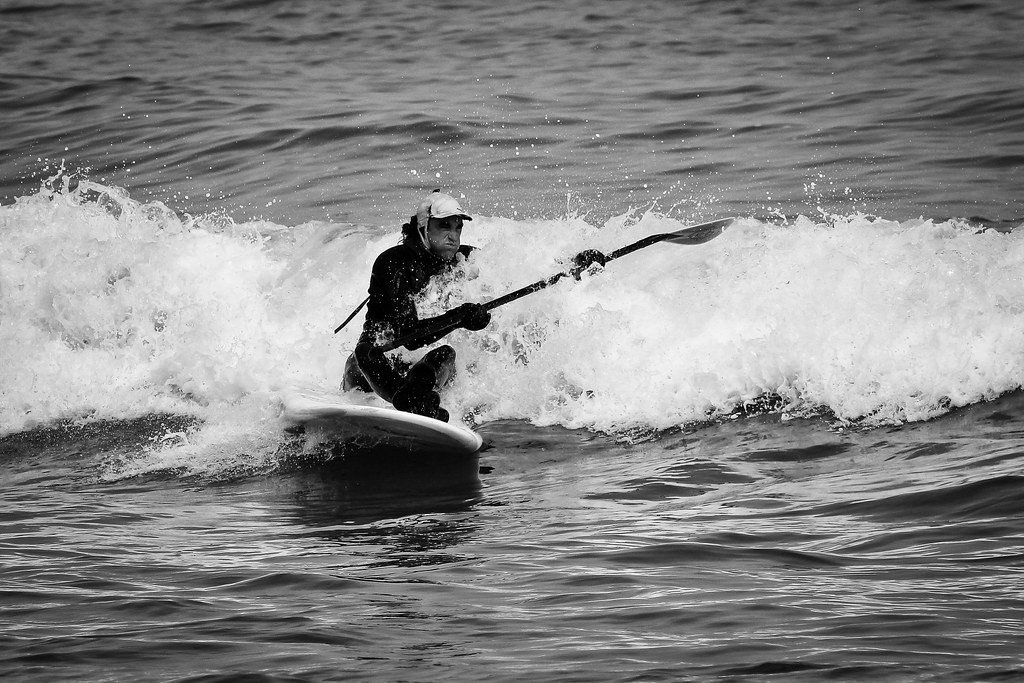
[446,303,491,331]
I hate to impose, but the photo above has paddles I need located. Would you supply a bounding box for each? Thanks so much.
[367,217,736,354]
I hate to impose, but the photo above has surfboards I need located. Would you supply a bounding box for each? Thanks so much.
[273,387,488,451]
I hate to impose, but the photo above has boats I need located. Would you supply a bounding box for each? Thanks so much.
[284,403,485,456]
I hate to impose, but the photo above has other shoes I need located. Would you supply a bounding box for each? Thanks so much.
[393,362,449,423]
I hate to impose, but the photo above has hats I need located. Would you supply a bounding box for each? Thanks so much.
[416,189,472,229]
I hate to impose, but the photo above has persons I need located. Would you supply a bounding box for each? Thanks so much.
[343,191,491,423]
